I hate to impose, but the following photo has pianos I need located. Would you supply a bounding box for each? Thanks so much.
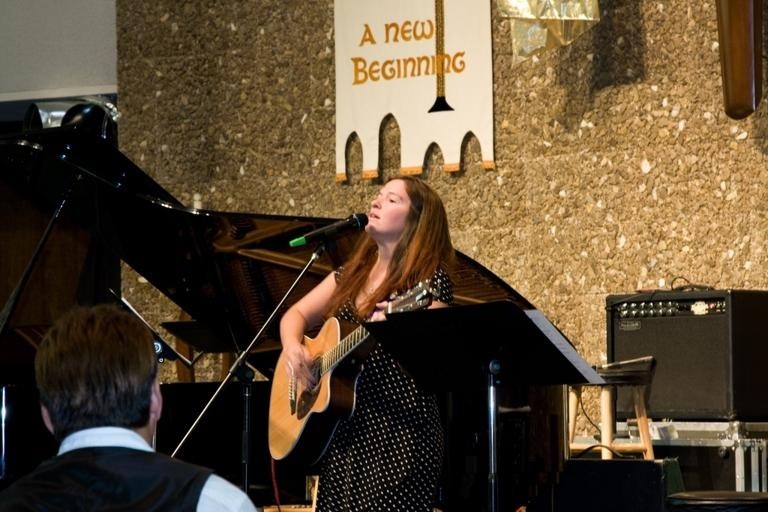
[1,128,669,508]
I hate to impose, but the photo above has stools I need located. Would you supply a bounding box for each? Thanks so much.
[565,356,656,462]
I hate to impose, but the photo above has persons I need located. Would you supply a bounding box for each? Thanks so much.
[278,176,453,511]
[0,304,258,511]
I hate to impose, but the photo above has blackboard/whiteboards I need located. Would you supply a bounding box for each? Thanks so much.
[0,0,118,102]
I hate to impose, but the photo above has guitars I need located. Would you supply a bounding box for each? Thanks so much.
[267,281,441,467]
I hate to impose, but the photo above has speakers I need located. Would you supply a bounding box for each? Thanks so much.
[560,457,687,512]
[604,287,768,421]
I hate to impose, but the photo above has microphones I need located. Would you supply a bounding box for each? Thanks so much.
[289,212,368,248]
